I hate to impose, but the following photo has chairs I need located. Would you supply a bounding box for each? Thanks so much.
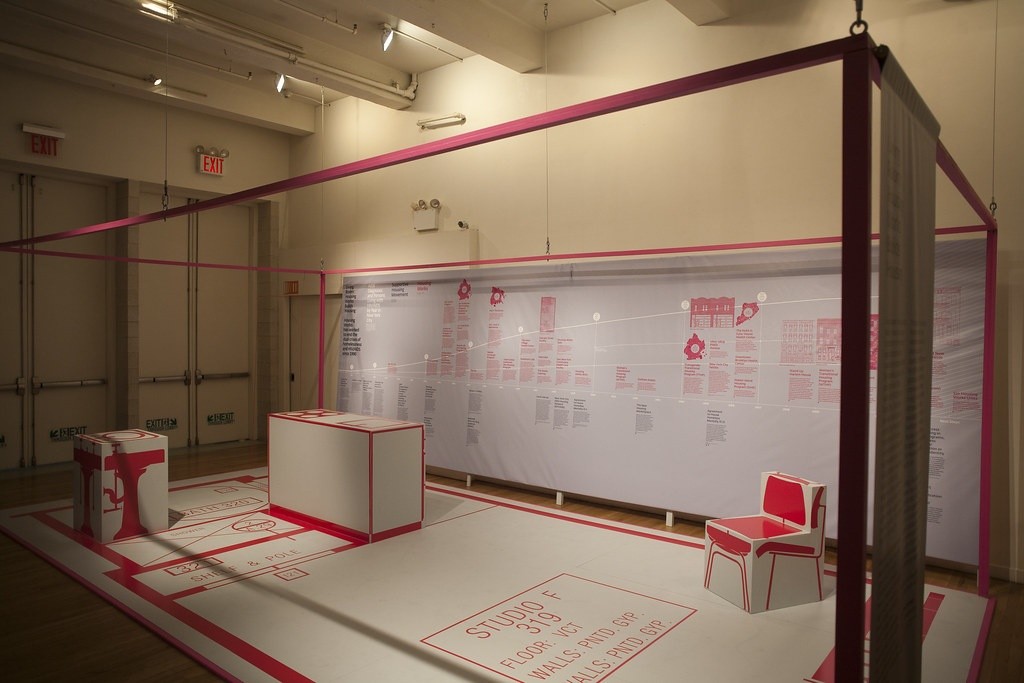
[702,471,826,613]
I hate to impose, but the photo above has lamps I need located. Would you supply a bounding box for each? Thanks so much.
[411,198,440,231]
[145,74,162,86]
[194,145,229,176]
[274,72,284,93]
[381,23,394,52]
